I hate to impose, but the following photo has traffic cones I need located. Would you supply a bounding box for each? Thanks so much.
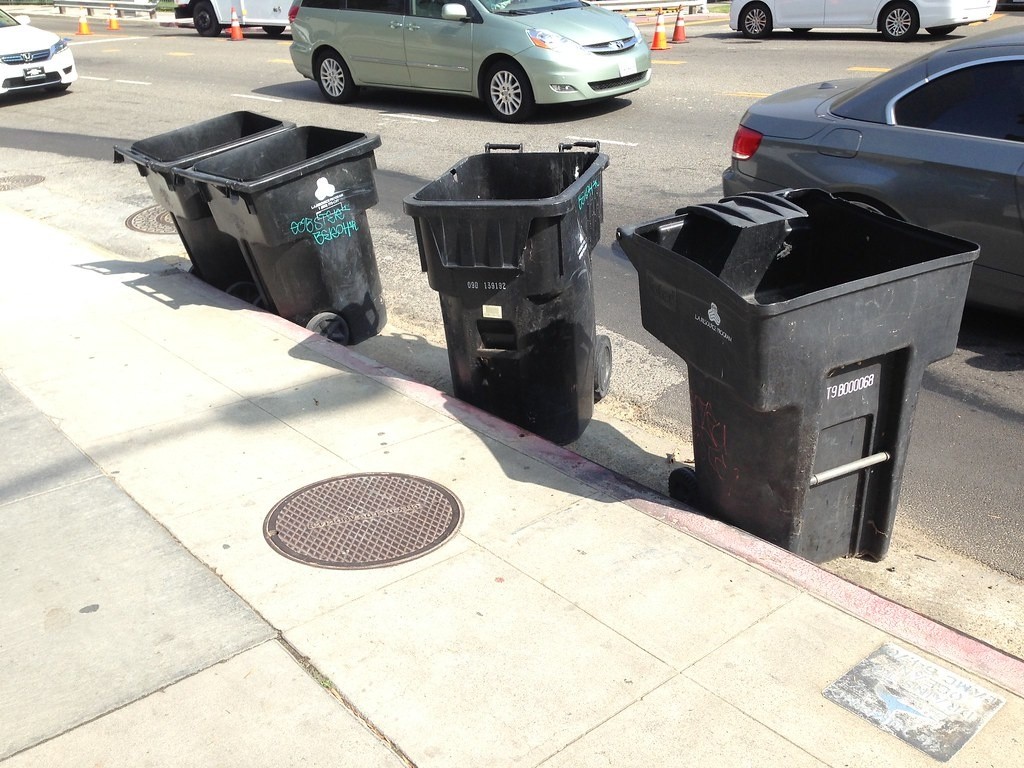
[666,5,690,44]
[649,7,672,49]
[106,4,121,30]
[76,5,94,35]
[229,6,245,42]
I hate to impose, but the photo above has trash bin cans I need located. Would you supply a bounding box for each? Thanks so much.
[170,125,387,347]
[113,109,298,305]
[616,189,982,562]
[403,141,614,447]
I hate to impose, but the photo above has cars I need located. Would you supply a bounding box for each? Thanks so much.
[174,0,294,38]
[722,25,1023,317]
[287,0,652,122]
[0,8,79,98]
[729,0,1000,41]
